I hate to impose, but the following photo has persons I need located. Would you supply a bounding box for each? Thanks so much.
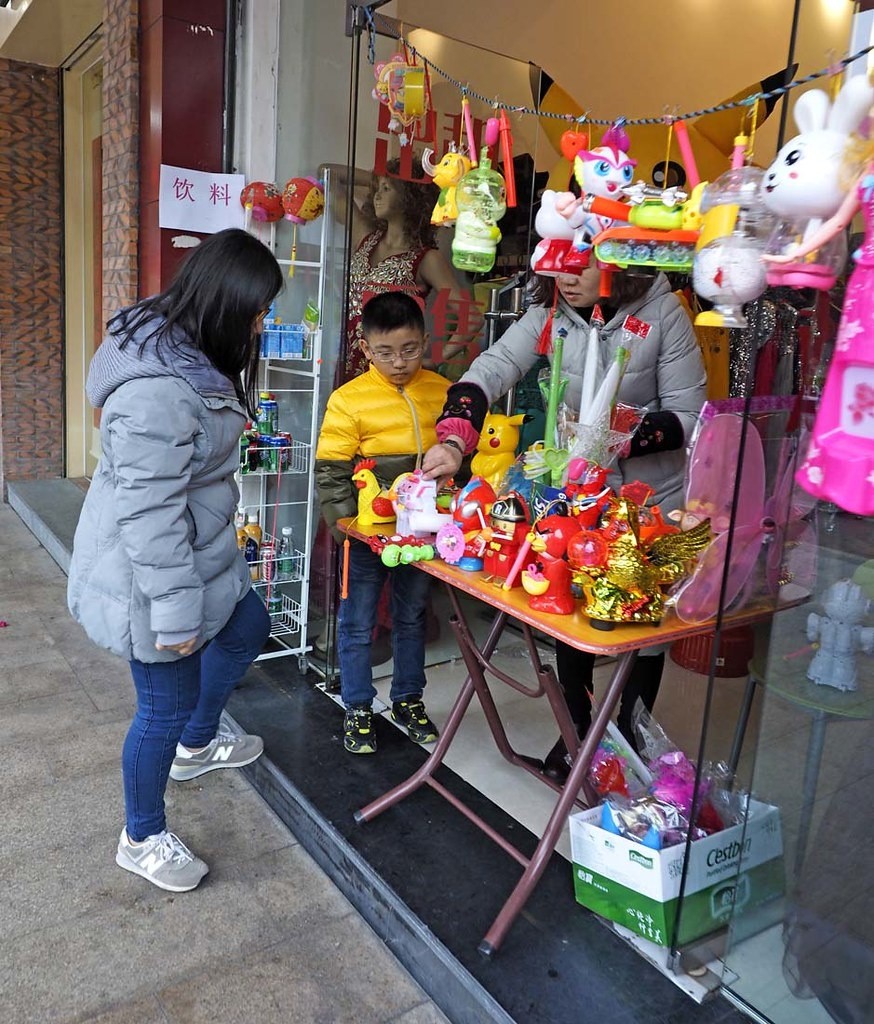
[342,157,485,383]
[421,234,708,786]
[314,290,454,752]
[68,229,284,892]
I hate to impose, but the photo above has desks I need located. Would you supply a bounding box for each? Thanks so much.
[335,513,814,959]
[723,635,874,895]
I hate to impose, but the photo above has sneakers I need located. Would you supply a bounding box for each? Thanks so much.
[391,698,440,744]
[169,723,264,781]
[116,824,209,892]
[343,705,378,753]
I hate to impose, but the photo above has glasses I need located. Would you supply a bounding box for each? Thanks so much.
[252,305,270,321]
[364,333,425,363]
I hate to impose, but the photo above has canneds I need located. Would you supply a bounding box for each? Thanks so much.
[259,540,276,582]
[252,400,292,474]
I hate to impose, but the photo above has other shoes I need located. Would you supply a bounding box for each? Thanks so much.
[543,724,588,779]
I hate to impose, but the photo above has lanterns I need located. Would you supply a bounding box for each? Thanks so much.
[282,176,325,278]
[240,182,284,240]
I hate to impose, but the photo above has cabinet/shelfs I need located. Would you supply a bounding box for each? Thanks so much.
[232,325,322,677]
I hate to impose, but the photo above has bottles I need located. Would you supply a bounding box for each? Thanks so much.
[240,392,275,474]
[234,507,295,622]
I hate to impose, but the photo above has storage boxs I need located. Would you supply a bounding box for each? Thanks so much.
[568,785,786,948]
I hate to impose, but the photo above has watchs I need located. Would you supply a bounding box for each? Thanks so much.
[440,440,463,454]
